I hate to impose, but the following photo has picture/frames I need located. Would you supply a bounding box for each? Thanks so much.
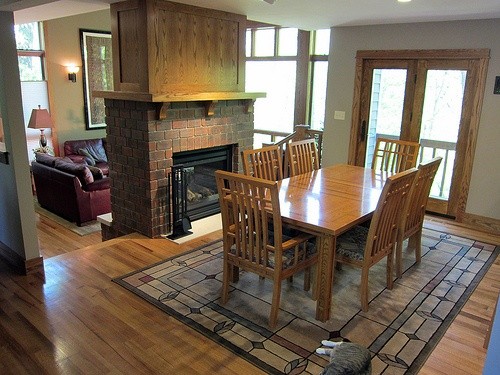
[79,27,113,130]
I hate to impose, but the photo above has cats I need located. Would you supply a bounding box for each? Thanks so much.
[315,339,372,375]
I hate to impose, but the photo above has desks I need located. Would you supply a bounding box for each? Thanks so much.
[218,164,400,323]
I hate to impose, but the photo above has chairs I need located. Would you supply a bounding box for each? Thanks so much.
[214,137,443,329]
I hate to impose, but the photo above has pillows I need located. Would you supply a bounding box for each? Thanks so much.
[34,139,108,184]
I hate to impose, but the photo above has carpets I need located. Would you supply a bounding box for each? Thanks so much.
[33,195,102,236]
[113,226,500,375]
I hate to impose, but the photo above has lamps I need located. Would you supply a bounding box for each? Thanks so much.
[68,67,79,83]
[28,104,55,147]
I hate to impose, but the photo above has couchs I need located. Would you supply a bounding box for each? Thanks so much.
[32,157,111,227]
[64,138,107,174]
